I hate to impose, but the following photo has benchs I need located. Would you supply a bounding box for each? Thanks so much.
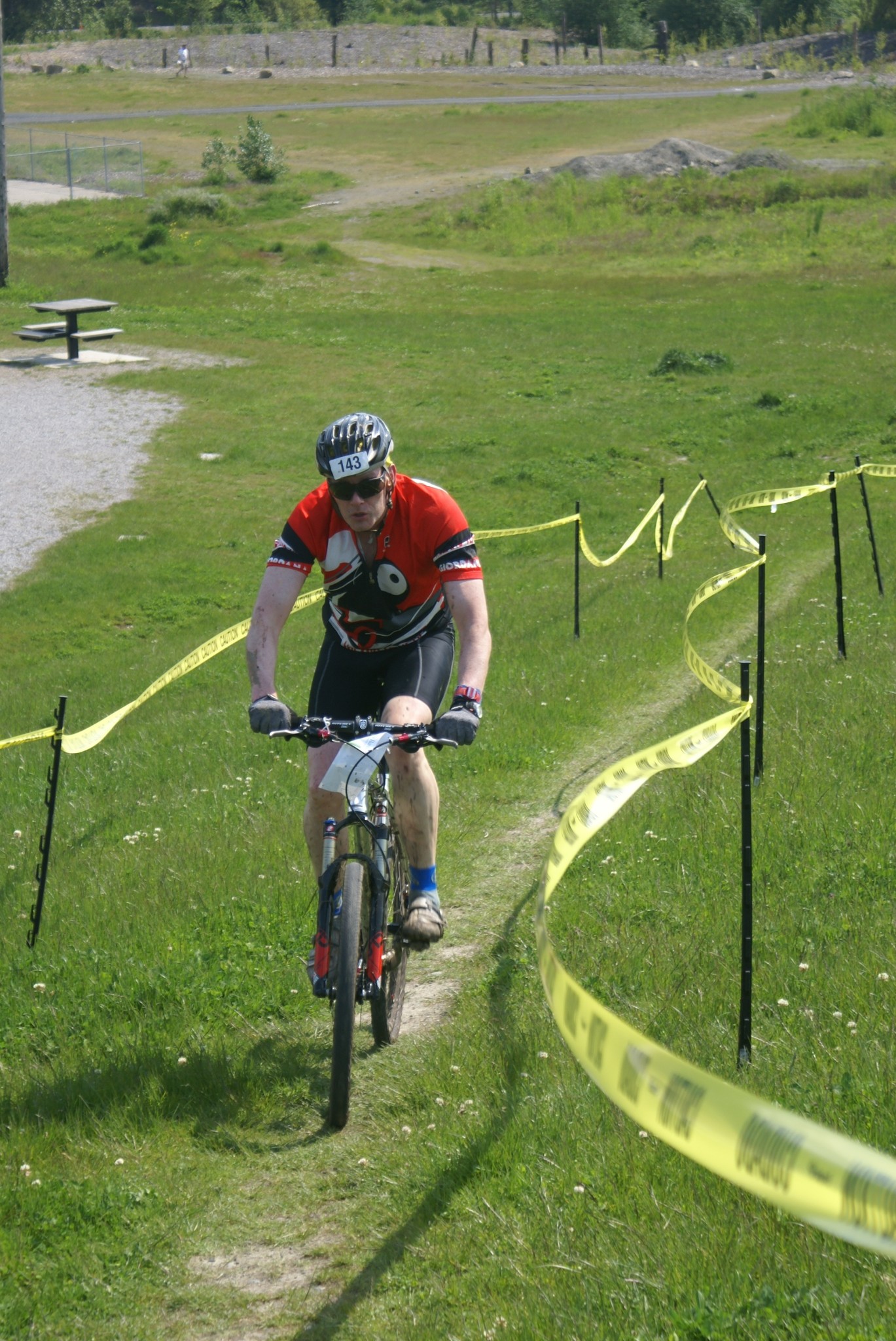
[10,330,56,342]
[68,327,124,341]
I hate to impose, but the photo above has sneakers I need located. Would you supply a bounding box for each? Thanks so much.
[399,896,444,940]
[307,945,334,984]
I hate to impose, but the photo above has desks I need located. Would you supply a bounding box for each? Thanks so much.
[25,297,119,359]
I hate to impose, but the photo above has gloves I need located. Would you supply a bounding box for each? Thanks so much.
[434,707,478,746]
[248,695,291,734]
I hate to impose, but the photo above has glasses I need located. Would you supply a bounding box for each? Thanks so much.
[327,470,386,501]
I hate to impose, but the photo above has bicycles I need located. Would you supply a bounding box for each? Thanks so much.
[268,714,459,1127]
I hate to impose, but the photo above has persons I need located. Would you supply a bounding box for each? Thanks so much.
[176,44,189,78]
[245,411,490,994]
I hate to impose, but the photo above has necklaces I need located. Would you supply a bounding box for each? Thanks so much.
[368,531,374,544]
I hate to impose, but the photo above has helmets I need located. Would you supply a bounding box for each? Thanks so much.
[316,412,391,478]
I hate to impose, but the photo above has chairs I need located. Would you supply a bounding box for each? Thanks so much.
[22,321,67,331]
